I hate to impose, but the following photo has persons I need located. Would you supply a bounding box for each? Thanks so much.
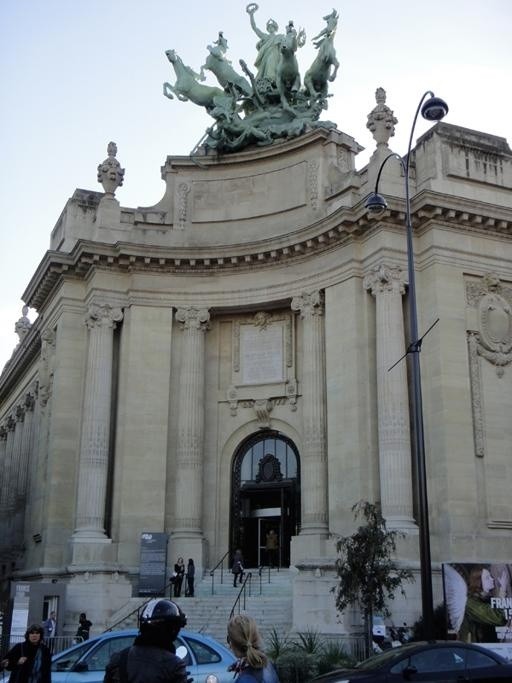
[2,622,52,683]
[265,528,279,568]
[183,558,195,597]
[465,565,509,643]
[245,2,285,86]
[72,612,92,644]
[102,597,194,682]
[232,548,244,587]
[173,556,185,595]
[41,610,57,657]
[226,616,281,682]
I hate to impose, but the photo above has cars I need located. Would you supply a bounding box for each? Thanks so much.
[311,640,511,682]
[2,629,242,682]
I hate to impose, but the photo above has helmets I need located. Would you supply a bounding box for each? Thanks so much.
[137,597,189,634]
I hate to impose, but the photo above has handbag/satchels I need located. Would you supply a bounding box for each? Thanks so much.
[231,562,242,574]
[169,573,179,584]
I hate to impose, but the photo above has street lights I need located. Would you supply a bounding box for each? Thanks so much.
[363,91,449,638]
